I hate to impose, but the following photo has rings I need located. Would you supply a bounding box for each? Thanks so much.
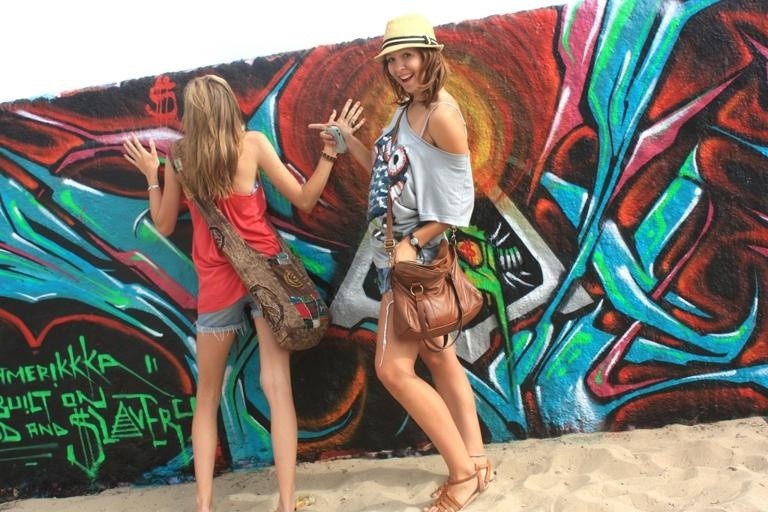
[351,119,356,127]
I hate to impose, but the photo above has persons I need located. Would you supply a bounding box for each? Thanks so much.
[123,74,368,512]
[307,15,495,512]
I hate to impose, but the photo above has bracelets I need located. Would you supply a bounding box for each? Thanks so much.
[321,151,338,162]
[147,184,159,191]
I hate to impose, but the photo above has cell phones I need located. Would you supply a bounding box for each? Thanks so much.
[325,125,347,154]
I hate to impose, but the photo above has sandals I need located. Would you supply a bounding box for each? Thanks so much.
[431,464,485,512]
[431,455,490,498]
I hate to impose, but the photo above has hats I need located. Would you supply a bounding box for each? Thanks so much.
[373,16,445,65]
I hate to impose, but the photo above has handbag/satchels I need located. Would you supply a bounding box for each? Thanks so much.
[389,239,485,353]
[228,239,330,351]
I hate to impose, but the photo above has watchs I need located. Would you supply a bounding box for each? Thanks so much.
[408,233,422,252]
